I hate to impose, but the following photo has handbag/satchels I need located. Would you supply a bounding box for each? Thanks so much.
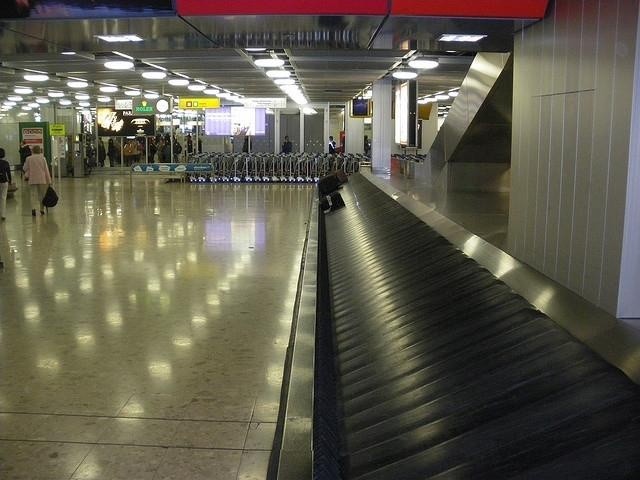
[42,187,58,207]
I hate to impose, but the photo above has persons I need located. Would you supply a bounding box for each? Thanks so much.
[242,136,252,152]
[329,136,336,153]
[282,136,292,153]
[23,145,51,216]
[364,135,370,154]
[0,148,11,220]
[97,135,202,167]
[19,141,32,181]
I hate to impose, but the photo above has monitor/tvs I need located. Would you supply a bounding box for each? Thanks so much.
[349,98,373,118]
